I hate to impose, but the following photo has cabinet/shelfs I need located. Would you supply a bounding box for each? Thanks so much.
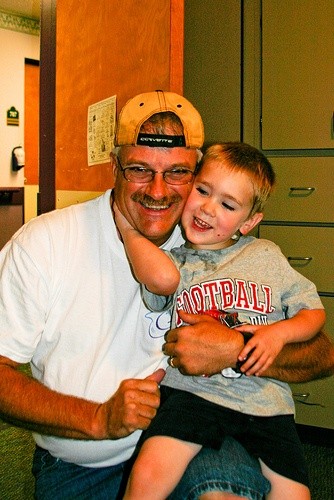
[182,0,333,428]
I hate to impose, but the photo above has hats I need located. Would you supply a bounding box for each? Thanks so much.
[114,89,205,149]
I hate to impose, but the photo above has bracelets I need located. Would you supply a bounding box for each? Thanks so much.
[219,330,255,378]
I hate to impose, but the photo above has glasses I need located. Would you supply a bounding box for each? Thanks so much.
[117,156,199,184]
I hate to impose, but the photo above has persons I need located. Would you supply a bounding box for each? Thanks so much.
[0,88,334,500]
[110,142,331,500]
